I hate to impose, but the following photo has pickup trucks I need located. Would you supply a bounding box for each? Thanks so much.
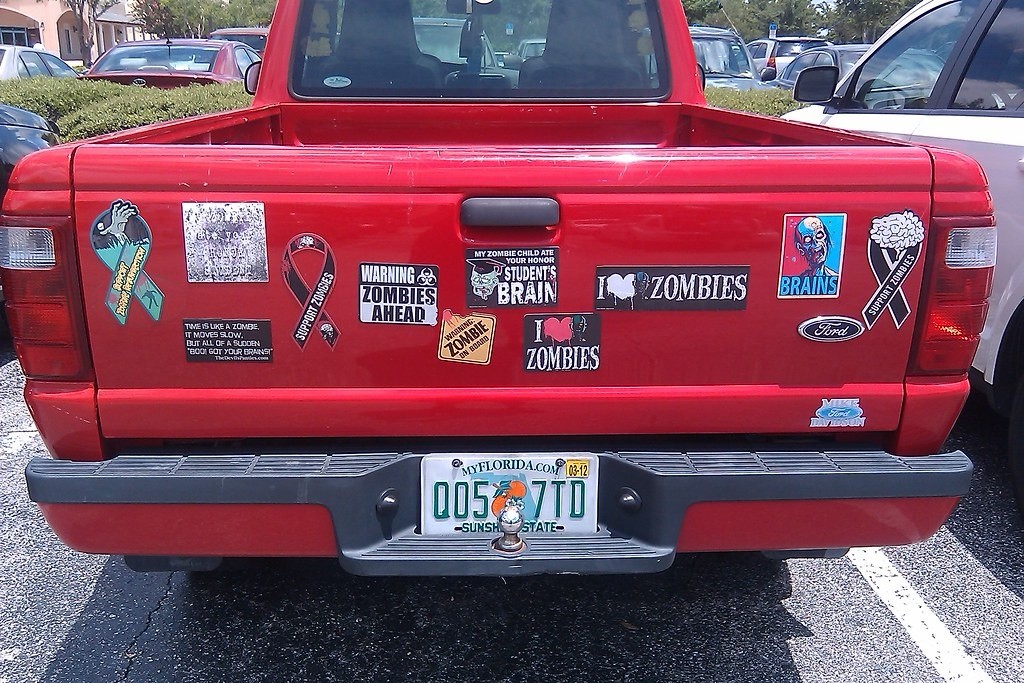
[0,0,998,578]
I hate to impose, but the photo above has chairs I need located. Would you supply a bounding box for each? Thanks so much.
[299,0,453,94]
[517,0,654,91]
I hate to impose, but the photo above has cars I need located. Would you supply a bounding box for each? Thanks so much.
[684,0,1024,486]
[1,41,83,81]
[74,37,262,88]
[207,28,269,58]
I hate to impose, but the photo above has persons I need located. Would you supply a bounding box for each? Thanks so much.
[33,41,45,54]
